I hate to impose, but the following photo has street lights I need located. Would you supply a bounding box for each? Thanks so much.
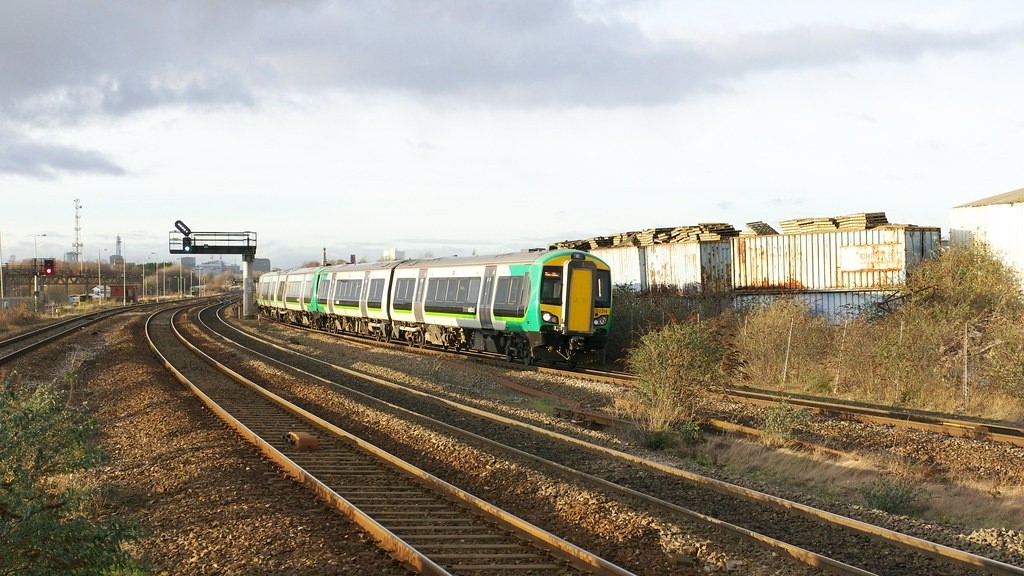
[117,242,125,306]
[143,257,150,298]
[164,256,200,297]
[98,248,107,305]
[35,234,47,274]
[152,252,158,304]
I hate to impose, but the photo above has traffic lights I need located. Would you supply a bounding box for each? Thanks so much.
[44,260,53,275]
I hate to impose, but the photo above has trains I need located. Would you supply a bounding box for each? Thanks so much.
[256,247,612,367]
